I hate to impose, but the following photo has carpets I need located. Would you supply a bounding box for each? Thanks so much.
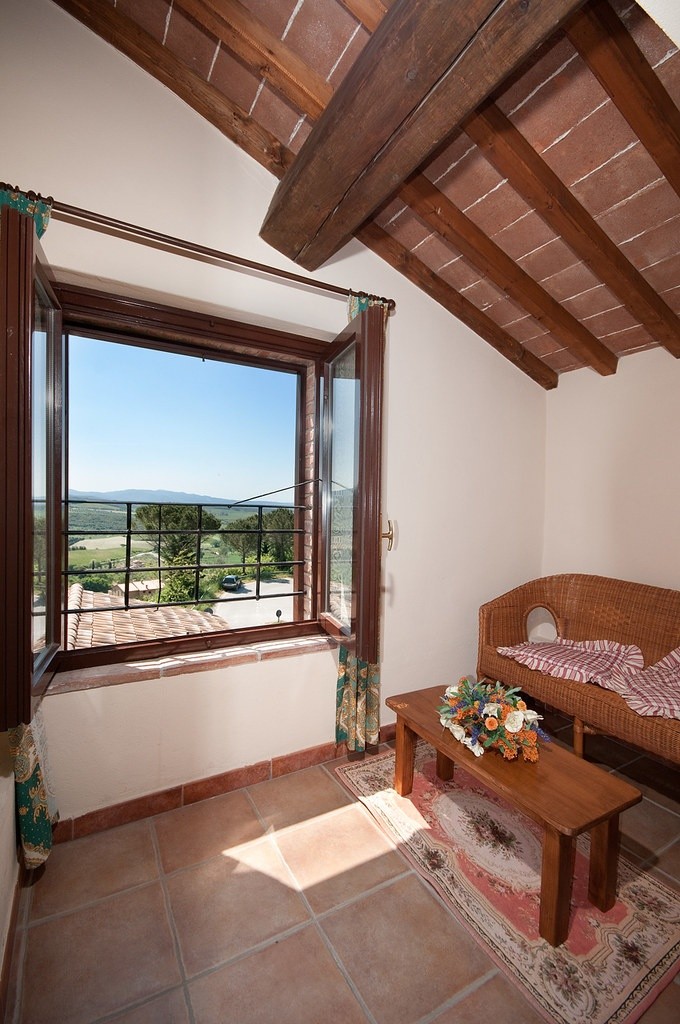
[319,738,680,1024]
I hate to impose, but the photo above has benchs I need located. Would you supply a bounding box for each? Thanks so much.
[478,572,680,764]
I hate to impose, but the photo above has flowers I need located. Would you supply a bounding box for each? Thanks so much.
[436,675,552,766]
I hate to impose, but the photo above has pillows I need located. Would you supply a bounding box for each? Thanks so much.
[499,634,646,685]
[610,645,679,720]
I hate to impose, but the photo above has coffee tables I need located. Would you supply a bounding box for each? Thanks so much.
[384,685,642,949]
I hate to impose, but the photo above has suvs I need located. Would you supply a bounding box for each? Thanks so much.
[222,575,241,591]
